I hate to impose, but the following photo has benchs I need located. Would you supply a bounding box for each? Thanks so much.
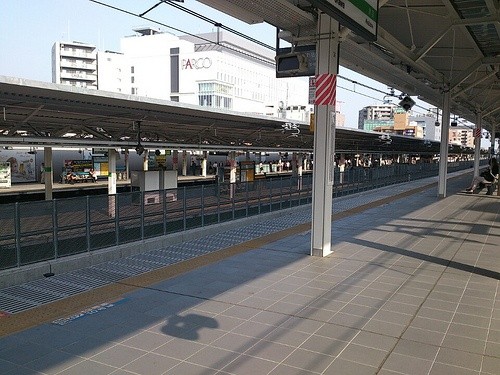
[483,174,499,195]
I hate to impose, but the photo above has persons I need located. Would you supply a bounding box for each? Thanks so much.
[191,161,196,176]
[209,161,224,174]
[336,155,416,184]
[89,168,97,182]
[278,160,292,172]
[465,158,499,193]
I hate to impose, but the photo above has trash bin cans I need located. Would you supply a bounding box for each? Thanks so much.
[216,166,225,183]
[152,167,178,204]
[130,169,160,206]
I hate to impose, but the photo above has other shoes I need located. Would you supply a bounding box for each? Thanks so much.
[467,191,473,194]
[466,188,472,191]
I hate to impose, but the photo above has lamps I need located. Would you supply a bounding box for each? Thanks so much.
[451,113,457,127]
[435,106,440,126]
[484,132,490,140]
